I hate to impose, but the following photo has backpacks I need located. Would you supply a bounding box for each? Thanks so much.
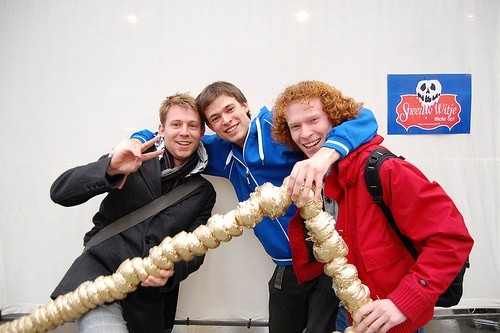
[365,148,469,307]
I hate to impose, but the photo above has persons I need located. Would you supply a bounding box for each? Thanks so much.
[107,81,381,333]
[272,81,474,333]
[49,94,217,333]
